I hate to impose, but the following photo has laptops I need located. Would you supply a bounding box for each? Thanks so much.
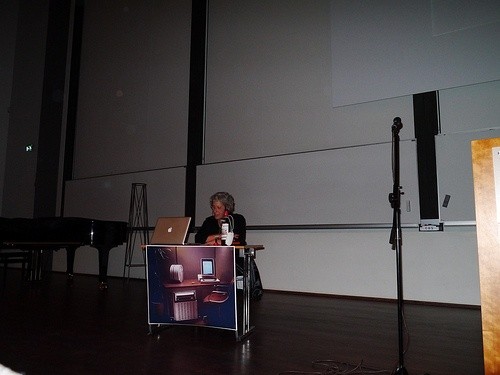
[150,217,201,246]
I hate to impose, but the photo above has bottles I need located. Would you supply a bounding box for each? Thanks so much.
[220,211,231,246]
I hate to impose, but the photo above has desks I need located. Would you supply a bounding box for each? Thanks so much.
[161,279,229,324]
[140,244,264,342]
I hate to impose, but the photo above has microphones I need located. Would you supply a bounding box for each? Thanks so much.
[392,117,402,126]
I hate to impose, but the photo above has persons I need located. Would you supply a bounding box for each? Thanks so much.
[195,192,246,245]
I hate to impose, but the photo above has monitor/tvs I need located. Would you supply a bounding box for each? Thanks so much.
[201,258,215,277]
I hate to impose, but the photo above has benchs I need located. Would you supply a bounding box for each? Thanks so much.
[0,249,36,304]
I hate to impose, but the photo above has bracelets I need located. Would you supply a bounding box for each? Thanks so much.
[215,240,217,244]
[215,235,217,239]
